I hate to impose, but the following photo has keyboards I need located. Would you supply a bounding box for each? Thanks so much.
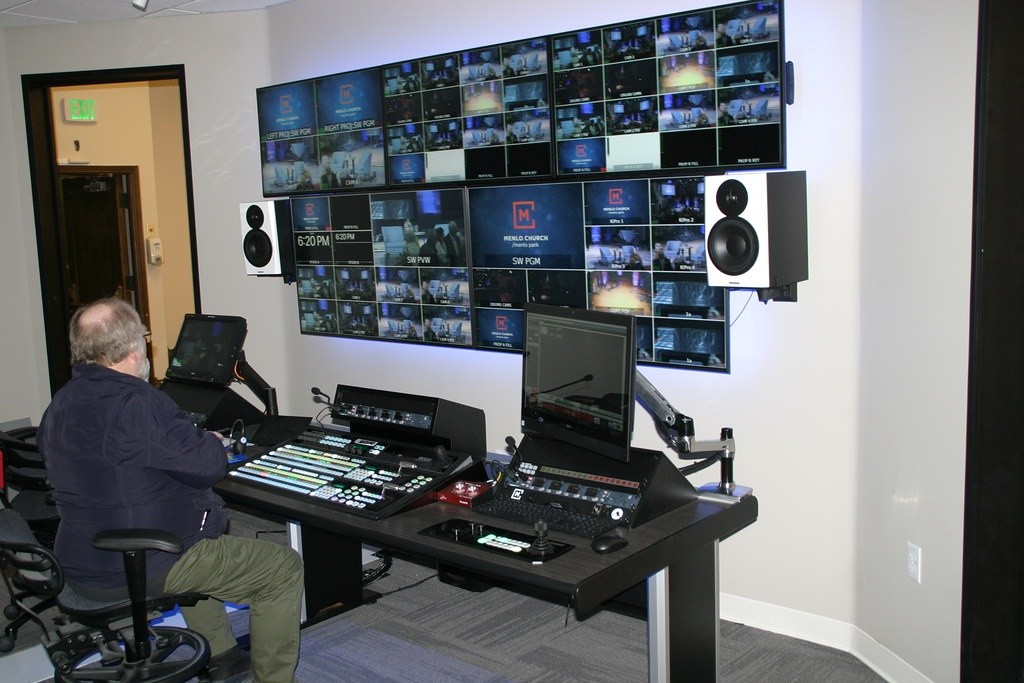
[472,496,620,539]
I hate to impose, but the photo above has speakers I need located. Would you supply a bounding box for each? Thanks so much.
[240,200,296,276]
[704,170,808,288]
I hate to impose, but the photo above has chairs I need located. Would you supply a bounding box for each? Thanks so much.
[0,426,210,683]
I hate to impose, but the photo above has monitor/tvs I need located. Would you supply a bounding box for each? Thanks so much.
[256,0,786,371]
[521,303,637,462]
[166,314,246,385]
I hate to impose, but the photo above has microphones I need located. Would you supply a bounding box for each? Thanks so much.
[311,387,337,407]
[505,436,523,462]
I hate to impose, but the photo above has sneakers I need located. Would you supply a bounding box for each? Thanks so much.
[197,646,255,683]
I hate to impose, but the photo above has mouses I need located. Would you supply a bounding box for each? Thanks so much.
[591,534,628,554]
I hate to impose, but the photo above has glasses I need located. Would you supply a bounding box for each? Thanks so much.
[142,330,153,344]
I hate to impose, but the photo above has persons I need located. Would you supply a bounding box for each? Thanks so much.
[36,297,304,683]
